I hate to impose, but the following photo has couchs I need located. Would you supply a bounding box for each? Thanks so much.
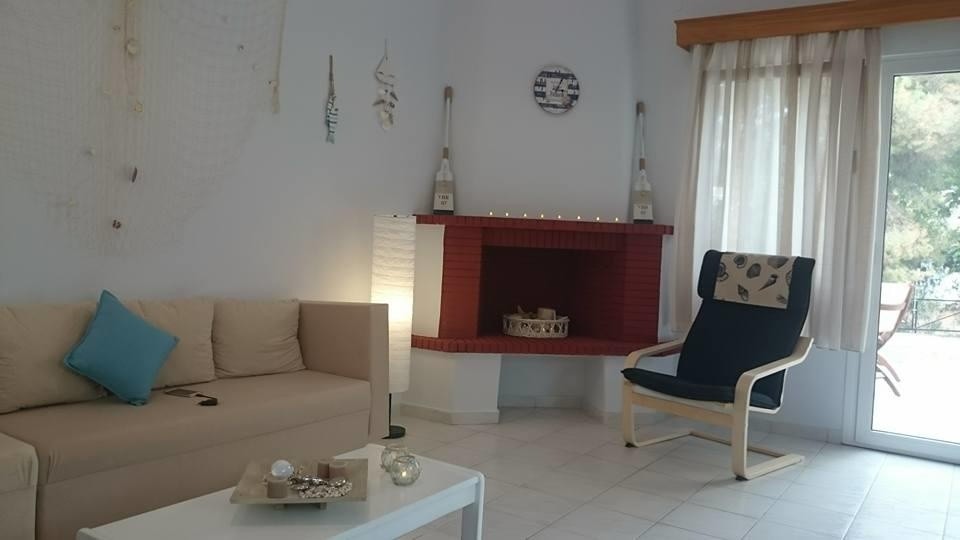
[0,299,390,540]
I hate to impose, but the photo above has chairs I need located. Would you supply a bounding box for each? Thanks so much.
[876,282,914,398]
[622,250,818,481]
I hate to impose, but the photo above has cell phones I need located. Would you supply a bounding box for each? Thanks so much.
[164,388,200,397]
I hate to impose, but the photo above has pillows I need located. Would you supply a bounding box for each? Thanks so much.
[67,288,183,407]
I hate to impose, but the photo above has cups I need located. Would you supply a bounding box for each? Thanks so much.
[381,443,410,475]
[390,456,421,486]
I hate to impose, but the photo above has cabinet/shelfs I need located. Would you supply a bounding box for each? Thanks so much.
[400,214,675,426]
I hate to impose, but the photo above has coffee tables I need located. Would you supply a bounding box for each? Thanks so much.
[74,440,485,539]
